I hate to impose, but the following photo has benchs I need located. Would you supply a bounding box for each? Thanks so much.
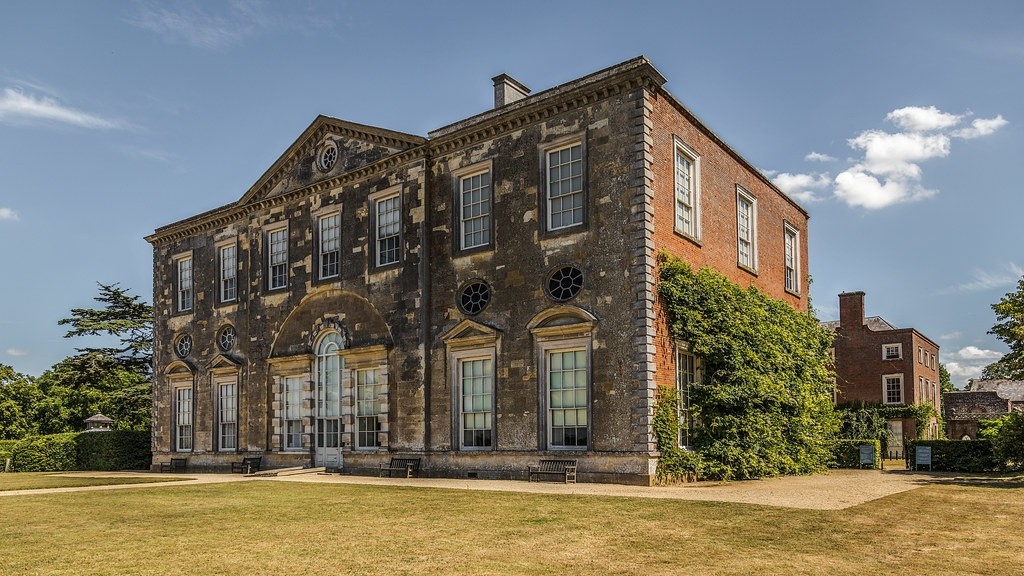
[526,459,578,484]
[379,458,421,478]
[230,456,262,474]
[160,458,187,473]
